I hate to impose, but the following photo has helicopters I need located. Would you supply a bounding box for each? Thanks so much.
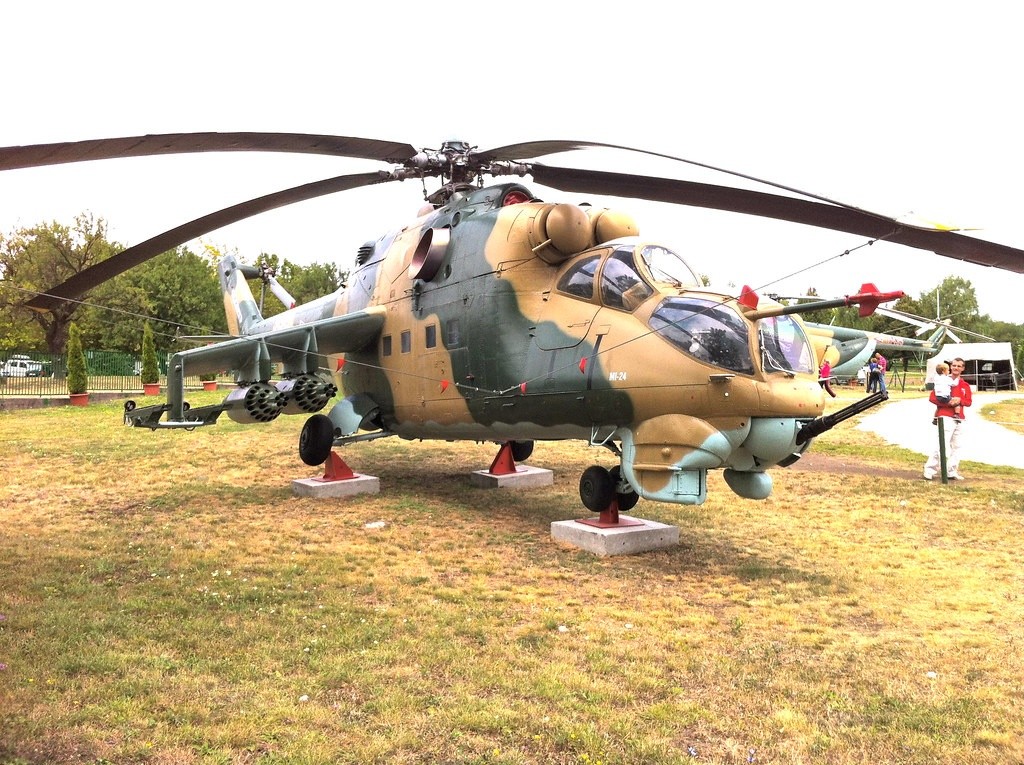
[0,133,1024,511]
[650,288,997,385]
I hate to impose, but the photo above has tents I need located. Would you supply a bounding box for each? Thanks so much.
[925,342,1018,391]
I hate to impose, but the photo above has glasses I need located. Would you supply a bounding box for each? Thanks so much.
[952,365,961,367]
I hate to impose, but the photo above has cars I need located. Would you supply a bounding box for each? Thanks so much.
[156,362,181,372]
[4,355,42,377]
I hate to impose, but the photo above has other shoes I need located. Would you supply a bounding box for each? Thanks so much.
[948,475,964,480]
[924,472,933,480]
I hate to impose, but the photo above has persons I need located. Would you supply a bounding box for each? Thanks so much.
[819,359,836,398]
[866,358,883,393]
[924,357,972,480]
[875,352,886,392]
[932,363,961,425]
